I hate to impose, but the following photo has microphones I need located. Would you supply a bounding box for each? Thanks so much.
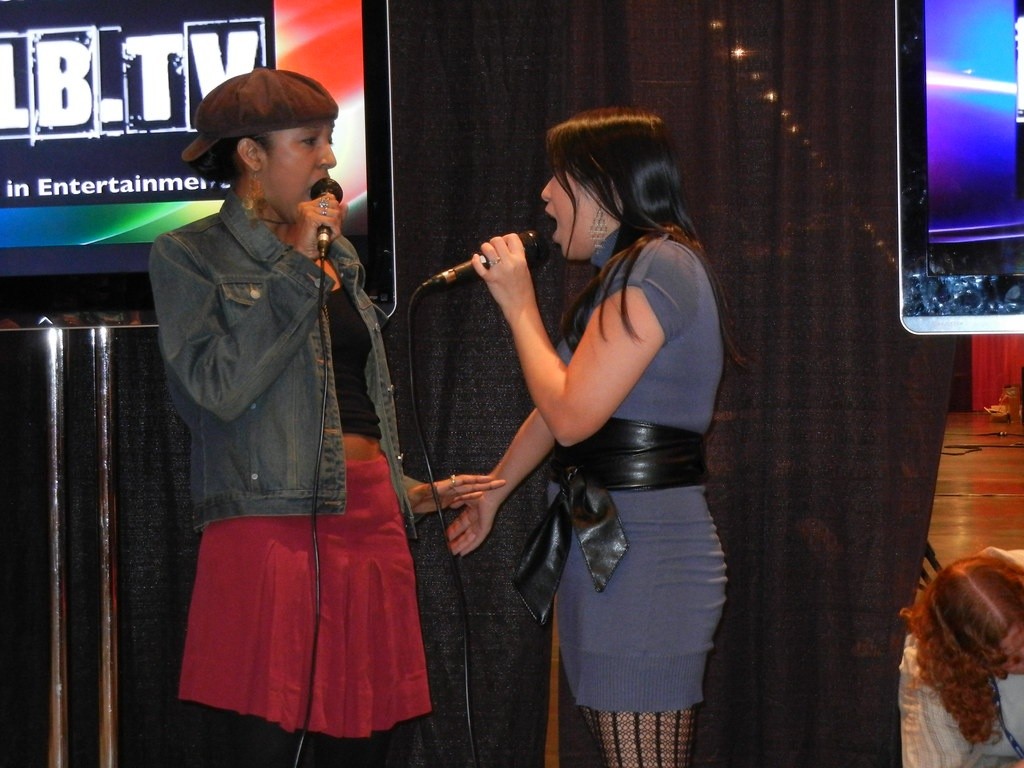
[311,177,344,260]
[423,231,541,289]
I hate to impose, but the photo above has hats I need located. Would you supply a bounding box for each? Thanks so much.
[178,67,339,164]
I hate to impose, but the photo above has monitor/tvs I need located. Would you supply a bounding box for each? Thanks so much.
[0,0,396,331]
[893,0,1024,336]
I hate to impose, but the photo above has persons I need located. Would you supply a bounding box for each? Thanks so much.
[150,66,508,768]
[446,107,755,768]
[899,545,1024,768]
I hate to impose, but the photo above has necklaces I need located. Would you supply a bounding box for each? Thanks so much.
[989,674,1024,758]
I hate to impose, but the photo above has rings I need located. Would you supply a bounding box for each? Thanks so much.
[319,198,329,207]
[321,208,327,215]
[489,257,500,266]
[450,474,456,486]
